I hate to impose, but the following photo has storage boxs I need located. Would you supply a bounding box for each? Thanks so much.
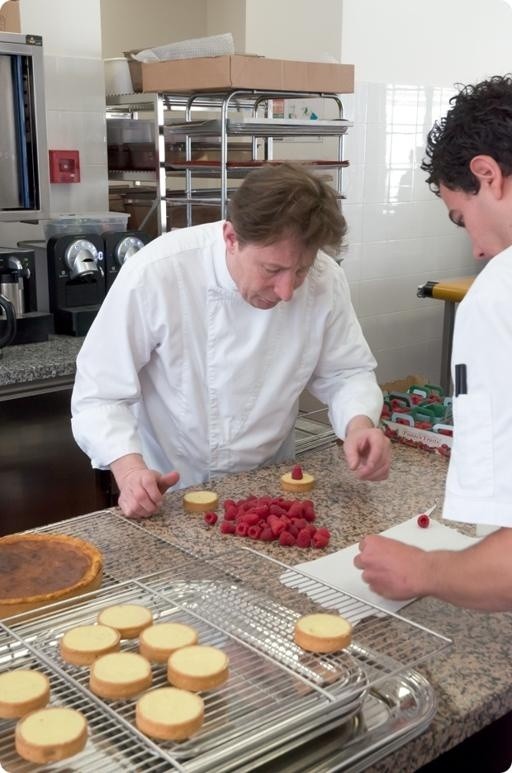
[122,51,355,95]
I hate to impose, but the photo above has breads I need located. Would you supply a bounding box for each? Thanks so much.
[167,644,228,692]
[183,490,219,512]
[62,624,121,664]
[296,613,352,653]
[140,623,199,661]
[136,687,204,740]
[0,670,49,718]
[0,534,103,618]
[97,604,153,639]
[281,472,314,492]
[16,707,88,764]
[90,653,152,697]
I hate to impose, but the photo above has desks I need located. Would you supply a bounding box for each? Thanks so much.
[418,277,475,396]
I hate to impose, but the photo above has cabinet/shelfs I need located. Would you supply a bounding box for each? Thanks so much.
[159,92,351,232]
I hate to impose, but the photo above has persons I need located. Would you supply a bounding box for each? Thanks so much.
[71,162,394,521]
[354,74,512,614]
